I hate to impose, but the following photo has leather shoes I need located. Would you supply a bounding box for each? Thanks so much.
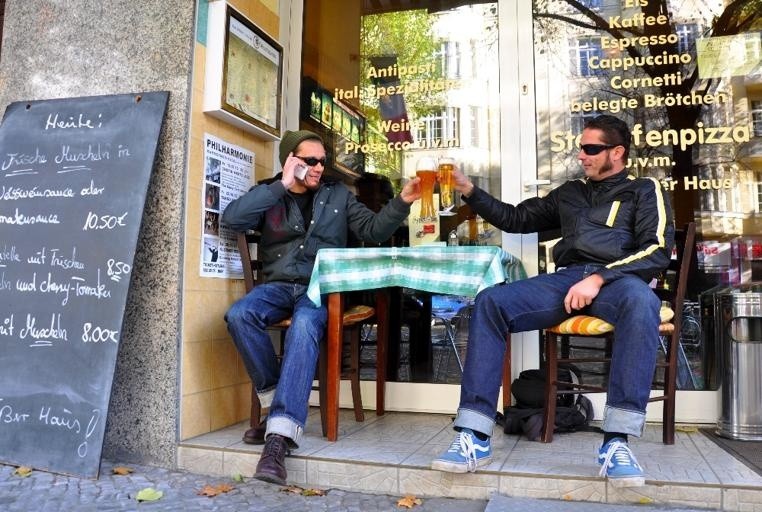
[253,435,288,486]
[242,420,266,444]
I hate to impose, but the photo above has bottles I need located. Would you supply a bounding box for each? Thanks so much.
[436,156,458,218]
[416,157,438,224]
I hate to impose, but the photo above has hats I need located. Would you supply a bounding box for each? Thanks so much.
[279,129,323,169]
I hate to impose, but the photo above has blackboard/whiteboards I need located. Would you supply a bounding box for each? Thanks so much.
[0,91,170,480]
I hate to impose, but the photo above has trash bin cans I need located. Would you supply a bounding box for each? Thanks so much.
[713,292,762,441]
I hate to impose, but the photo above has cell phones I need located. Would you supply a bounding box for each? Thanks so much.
[293,163,309,181]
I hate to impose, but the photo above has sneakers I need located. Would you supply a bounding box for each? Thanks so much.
[431,428,493,475]
[597,437,645,488]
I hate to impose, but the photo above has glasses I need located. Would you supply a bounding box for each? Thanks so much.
[579,143,617,155]
[294,156,327,167]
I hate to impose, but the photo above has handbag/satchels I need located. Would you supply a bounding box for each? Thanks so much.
[510,367,581,408]
[503,396,594,442]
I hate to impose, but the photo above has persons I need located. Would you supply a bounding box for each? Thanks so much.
[219,129,424,487]
[351,165,479,393]
[430,112,676,490]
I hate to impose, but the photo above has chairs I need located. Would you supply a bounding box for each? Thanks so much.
[237,230,376,437]
[539,221,698,446]
